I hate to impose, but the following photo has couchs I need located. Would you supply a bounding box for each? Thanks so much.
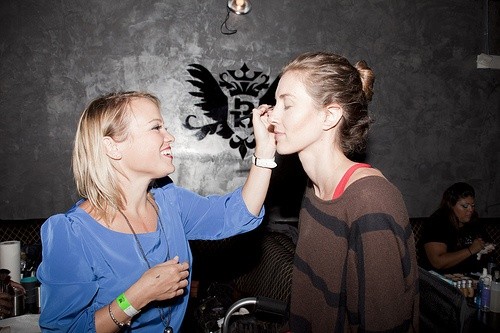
[0,217,48,247]
[408,216,500,274]
[193,224,297,333]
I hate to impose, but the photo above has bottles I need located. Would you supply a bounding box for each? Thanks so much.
[453,279,474,297]
[480,268,492,311]
[489,270,500,313]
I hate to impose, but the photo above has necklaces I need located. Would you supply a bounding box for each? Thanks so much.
[118,199,174,333]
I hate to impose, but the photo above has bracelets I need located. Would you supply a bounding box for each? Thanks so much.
[467,247,472,255]
[253,153,277,168]
[116,294,141,318]
[108,304,130,328]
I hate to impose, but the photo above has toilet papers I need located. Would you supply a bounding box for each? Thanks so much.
[0,240,23,284]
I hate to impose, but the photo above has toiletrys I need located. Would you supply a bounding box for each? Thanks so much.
[444,262,500,314]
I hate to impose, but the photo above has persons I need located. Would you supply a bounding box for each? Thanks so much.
[270,52,421,333]
[420,182,495,276]
[36,90,277,333]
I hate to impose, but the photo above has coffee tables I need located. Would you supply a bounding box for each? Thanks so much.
[440,273,500,333]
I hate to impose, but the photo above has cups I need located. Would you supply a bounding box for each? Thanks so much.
[11,291,24,317]
